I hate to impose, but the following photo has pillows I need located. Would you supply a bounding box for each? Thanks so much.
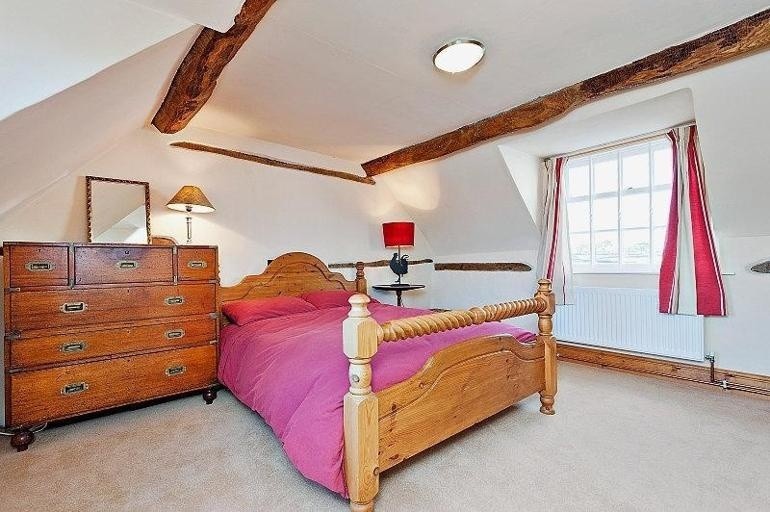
[221,291,378,327]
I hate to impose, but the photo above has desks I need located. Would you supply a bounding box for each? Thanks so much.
[373,286,425,307]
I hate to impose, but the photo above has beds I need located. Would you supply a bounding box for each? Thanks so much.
[221,252,557,512]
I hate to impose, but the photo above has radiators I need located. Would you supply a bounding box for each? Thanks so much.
[557,286,705,362]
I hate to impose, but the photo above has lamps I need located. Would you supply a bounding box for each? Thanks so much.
[165,186,217,245]
[382,222,414,287]
[433,39,486,73]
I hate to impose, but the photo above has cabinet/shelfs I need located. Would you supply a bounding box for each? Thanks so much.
[3,242,220,452]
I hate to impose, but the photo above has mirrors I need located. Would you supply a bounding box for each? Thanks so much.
[86,176,152,245]
